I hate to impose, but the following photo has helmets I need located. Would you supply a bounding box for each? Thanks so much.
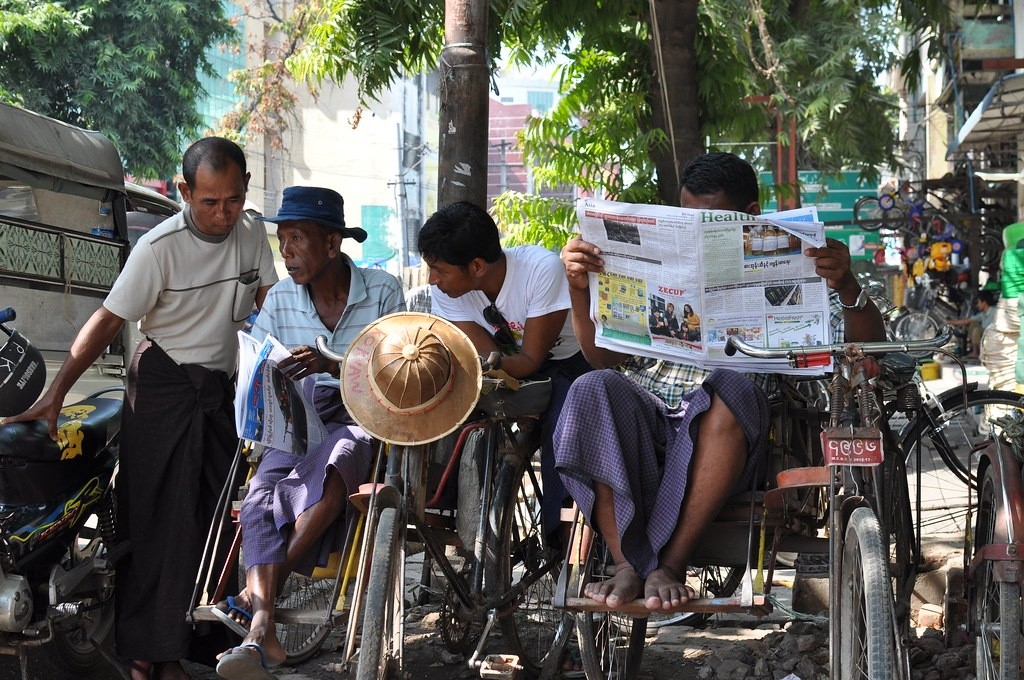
[0,328,47,417]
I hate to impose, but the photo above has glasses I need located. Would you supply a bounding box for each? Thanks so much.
[483,303,517,350]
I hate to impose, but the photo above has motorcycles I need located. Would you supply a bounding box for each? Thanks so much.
[0,304,132,680]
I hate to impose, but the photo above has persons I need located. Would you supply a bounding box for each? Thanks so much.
[561,152,887,612]
[419,201,606,583]
[649,303,701,342]
[1,138,278,679]
[210,186,407,679]
[946,292,997,359]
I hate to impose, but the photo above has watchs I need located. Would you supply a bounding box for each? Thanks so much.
[837,287,869,309]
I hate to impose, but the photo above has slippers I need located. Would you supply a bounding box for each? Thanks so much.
[210,596,254,638]
[216,643,279,680]
[960,355,979,359]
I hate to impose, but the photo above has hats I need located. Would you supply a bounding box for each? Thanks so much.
[339,311,483,445]
[253,186,368,242]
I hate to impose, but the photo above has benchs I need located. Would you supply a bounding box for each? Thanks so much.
[718,466,806,532]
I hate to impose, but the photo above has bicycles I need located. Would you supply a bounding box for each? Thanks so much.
[853,147,1007,359]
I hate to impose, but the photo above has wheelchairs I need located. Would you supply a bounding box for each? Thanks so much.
[183,331,1024,680]
